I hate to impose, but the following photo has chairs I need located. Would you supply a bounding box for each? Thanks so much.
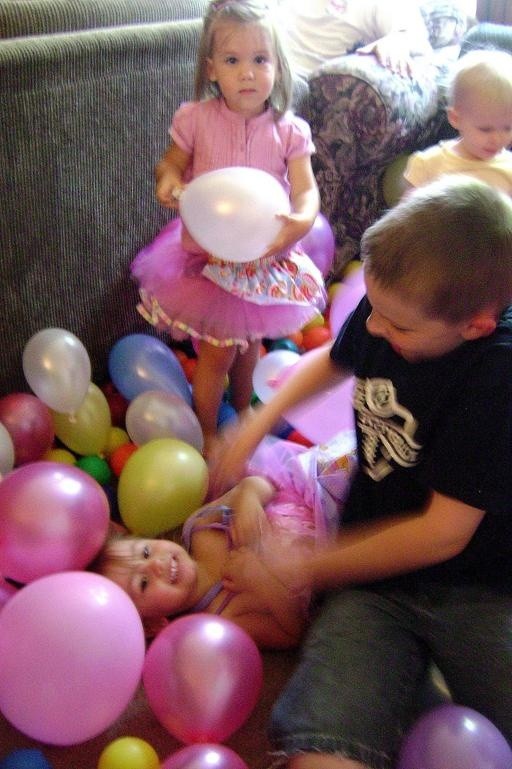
[0,0,512,394]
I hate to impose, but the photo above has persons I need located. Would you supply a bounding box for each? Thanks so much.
[399,49,511,199]
[207,172,511,768]
[132,0,329,433]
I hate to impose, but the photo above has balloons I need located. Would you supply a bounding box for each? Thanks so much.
[0,209,365,769]
[396,703,511,768]
[173,164,289,266]
